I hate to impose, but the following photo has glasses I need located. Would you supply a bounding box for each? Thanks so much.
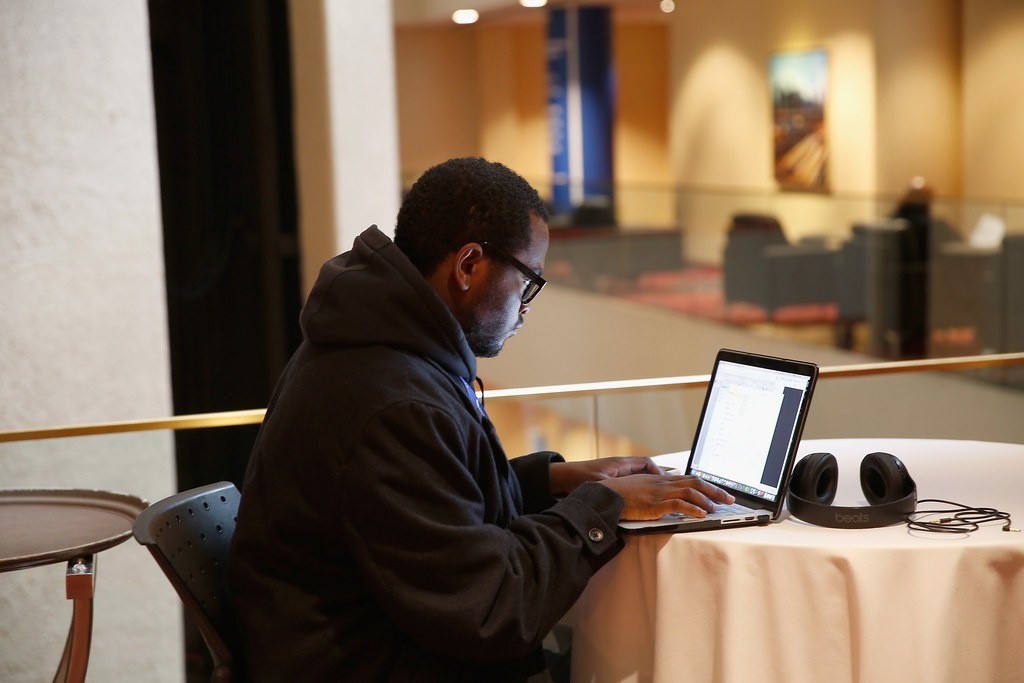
[451,238,548,305]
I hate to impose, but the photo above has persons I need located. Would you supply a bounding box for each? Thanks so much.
[225,156,735,683]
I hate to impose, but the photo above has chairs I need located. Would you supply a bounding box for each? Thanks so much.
[131,481,253,683]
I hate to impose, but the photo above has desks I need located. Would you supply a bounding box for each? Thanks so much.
[569,438,1024,683]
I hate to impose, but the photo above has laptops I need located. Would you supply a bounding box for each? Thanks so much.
[616,348,819,535]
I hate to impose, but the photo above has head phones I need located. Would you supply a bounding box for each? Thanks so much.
[786,453,919,529]
